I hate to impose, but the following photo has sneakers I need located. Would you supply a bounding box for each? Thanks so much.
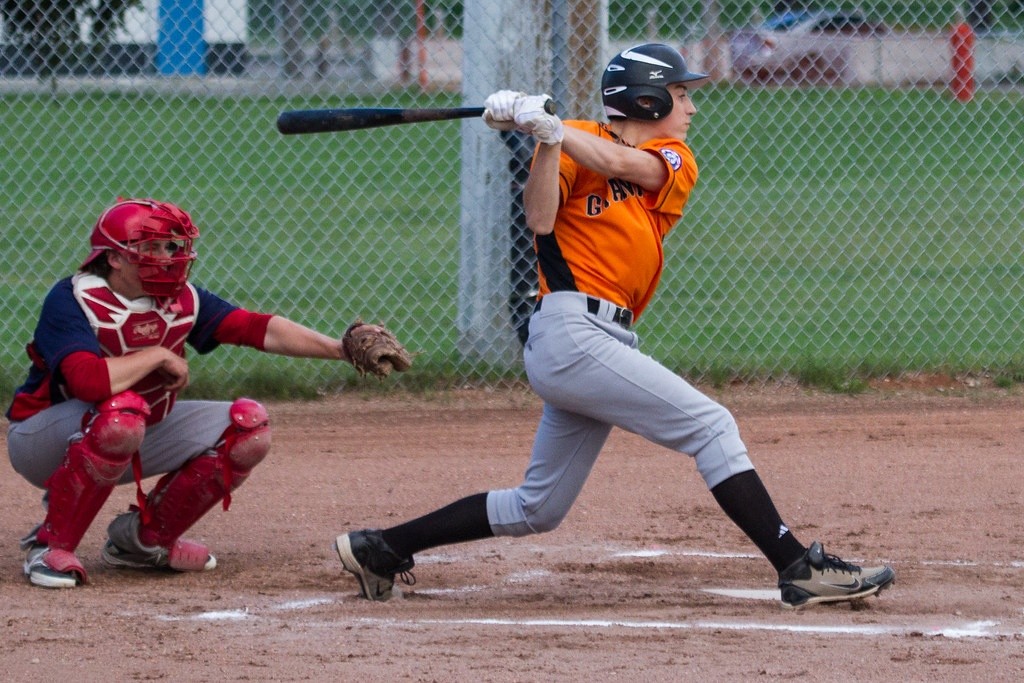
[779,540,897,608]
[334,530,417,601]
[24,536,80,590]
[103,531,220,573]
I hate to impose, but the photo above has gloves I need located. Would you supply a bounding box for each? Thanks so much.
[482,91,565,147]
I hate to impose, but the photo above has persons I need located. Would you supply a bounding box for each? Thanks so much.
[334,44,894,611]
[5,197,412,587]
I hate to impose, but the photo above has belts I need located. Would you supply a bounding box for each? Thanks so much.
[587,297,635,330]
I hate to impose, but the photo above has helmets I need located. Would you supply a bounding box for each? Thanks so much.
[602,42,707,124]
[78,201,171,272]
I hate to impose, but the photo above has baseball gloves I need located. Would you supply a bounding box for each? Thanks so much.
[340,317,413,380]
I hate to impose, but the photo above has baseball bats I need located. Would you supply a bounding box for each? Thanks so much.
[275,95,558,135]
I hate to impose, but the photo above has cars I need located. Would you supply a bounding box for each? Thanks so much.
[725,8,896,87]
